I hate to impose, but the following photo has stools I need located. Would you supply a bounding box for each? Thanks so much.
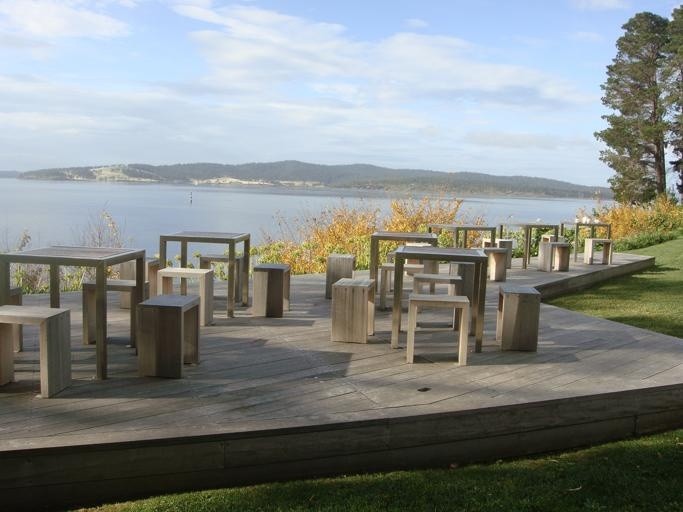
[543,235,565,243]
[155,265,215,330]
[385,252,418,292]
[250,263,293,319]
[326,255,356,300]
[80,278,152,347]
[584,238,613,265]
[485,246,508,283]
[407,292,471,368]
[497,283,543,353]
[197,249,246,305]
[328,275,377,345]
[482,238,512,269]
[118,256,162,311]
[378,263,425,311]
[134,293,203,381]
[9,285,26,353]
[0,304,77,400]
[412,271,463,332]
[539,241,571,272]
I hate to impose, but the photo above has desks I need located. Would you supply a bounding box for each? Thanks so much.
[158,230,252,320]
[390,245,488,355]
[561,221,612,262]
[1,244,149,384]
[427,223,498,249]
[498,223,559,270]
[369,231,439,295]
